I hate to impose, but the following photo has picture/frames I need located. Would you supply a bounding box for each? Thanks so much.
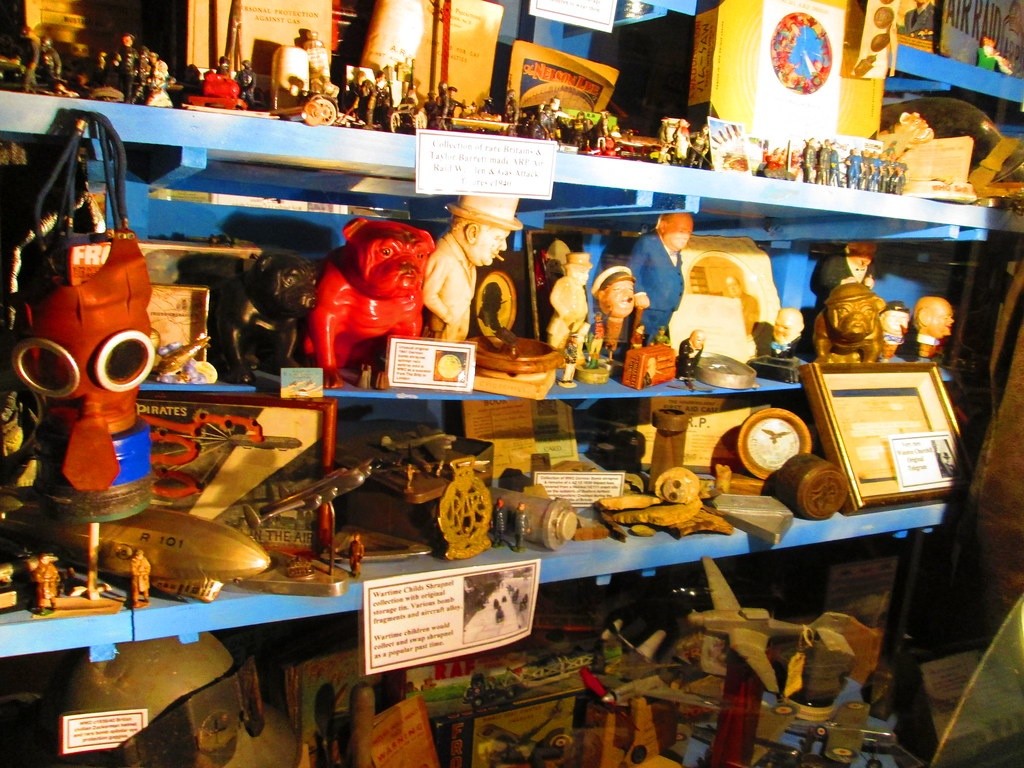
[796,361,961,515]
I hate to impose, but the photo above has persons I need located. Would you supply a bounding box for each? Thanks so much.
[629,213,693,350]
[490,499,530,551]
[912,296,955,361]
[217,57,257,107]
[879,303,908,361]
[96,33,175,107]
[521,97,911,194]
[290,70,391,131]
[18,26,65,95]
[34,555,59,614]
[481,283,502,330]
[546,252,604,384]
[129,550,151,607]
[422,194,523,341]
[591,266,642,363]
[349,535,364,580]
[771,309,805,358]
[426,82,519,136]
[820,240,875,289]
[679,330,705,381]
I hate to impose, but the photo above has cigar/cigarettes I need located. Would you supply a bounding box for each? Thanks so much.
[495,254,504,261]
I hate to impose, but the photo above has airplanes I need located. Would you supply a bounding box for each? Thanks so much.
[688,556,850,694]
[750,702,898,768]
[601,619,722,711]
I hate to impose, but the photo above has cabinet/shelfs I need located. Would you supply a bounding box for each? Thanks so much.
[0,0,1024,768]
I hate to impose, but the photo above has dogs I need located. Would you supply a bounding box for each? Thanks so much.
[210,255,318,385]
[303,217,435,388]
[813,284,884,365]
